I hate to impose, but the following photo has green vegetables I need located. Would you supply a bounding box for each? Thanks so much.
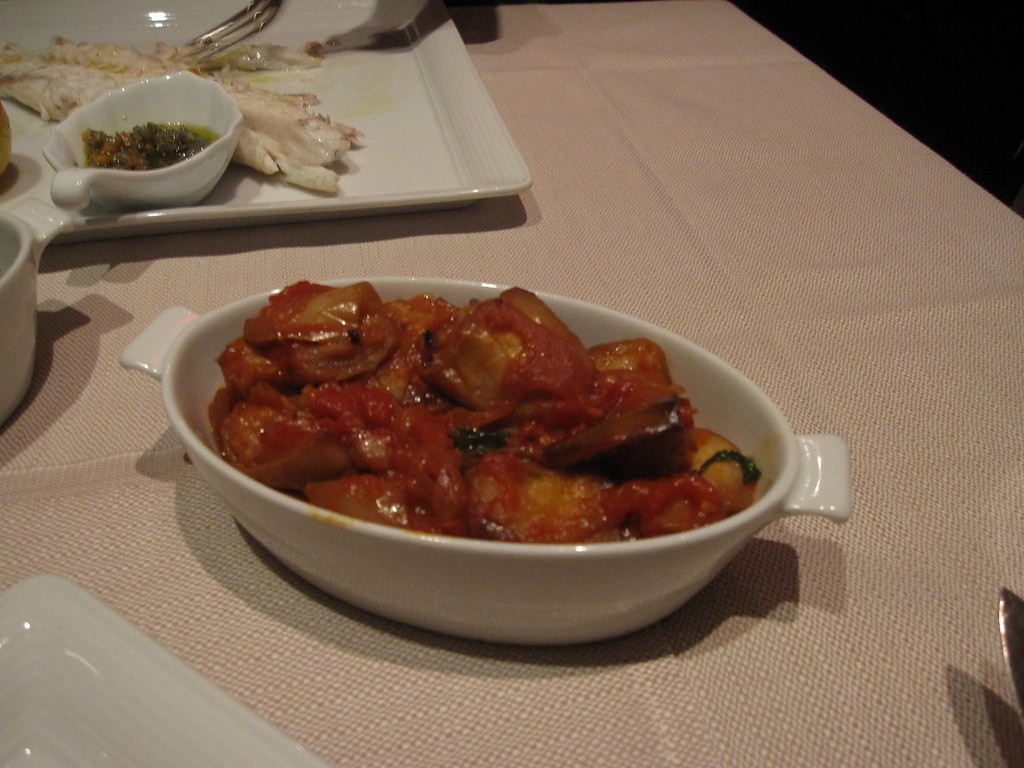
[420,331,506,456]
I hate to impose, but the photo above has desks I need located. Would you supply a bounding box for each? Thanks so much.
[0,0,1024,768]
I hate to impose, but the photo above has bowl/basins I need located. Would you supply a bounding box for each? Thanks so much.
[0,197,71,437]
[41,69,245,214]
[116,275,854,647]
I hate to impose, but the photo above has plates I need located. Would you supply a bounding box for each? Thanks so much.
[1,0,532,245]
[0,571,332,768]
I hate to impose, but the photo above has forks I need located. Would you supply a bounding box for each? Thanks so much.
[180,0,287,68]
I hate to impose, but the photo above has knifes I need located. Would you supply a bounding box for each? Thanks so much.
[999,586,1024,716]
